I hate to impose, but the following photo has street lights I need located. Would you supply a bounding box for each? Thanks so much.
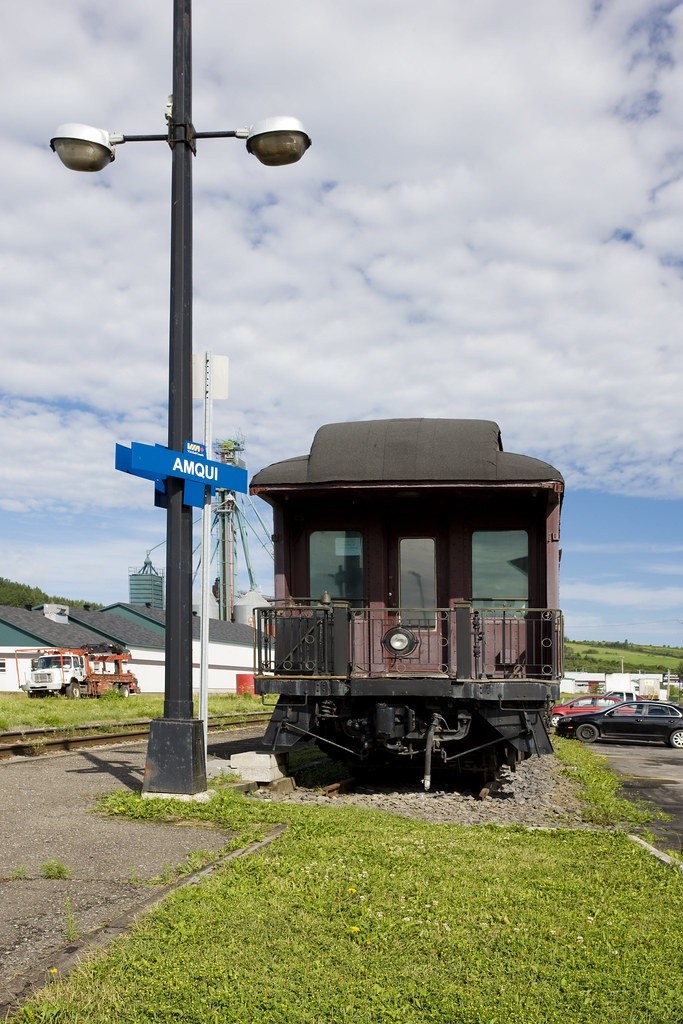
[50,114,312,794]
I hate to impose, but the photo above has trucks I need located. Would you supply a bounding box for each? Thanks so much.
[15,639,141,700]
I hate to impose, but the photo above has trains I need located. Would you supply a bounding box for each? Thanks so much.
[248,416,566,790]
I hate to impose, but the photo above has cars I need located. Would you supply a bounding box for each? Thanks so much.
[575,690,650,707]
[557,699,683,749]
[548,695,638,728]
